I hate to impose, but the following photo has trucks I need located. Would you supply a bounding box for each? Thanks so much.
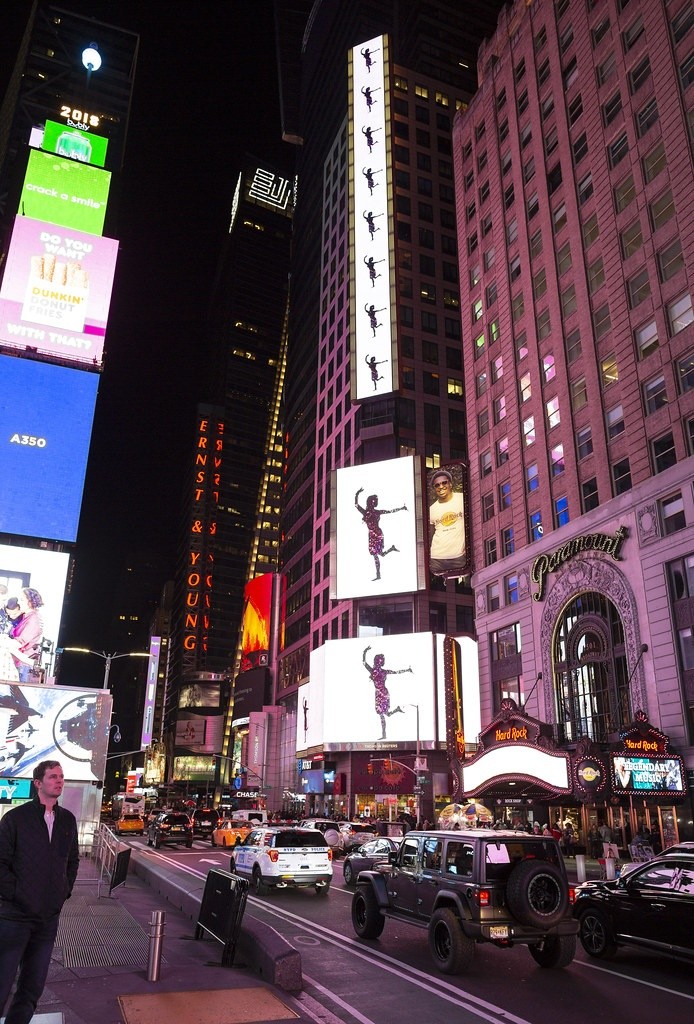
[231,810,268,827]
[110,791,145,819]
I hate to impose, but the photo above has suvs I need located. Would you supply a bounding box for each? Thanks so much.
[350,830,581,974]
[569,842,694,965]
[293,818,342,859]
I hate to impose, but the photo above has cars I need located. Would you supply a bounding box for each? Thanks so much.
[113,808,474,896]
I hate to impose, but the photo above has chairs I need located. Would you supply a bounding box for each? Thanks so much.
[456,855,475,875]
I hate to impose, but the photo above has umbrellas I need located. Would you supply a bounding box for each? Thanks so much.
[440,801,492,829]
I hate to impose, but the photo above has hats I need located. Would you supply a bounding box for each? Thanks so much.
[542,825,546,829]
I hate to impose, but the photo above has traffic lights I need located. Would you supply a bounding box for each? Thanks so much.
[146,740,159,759]
[367,763,373,774]
[212,757,216,766]
[113,769,119,781]
[384,759,390,771]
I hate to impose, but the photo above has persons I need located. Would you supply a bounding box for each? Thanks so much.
[183,722,196,743]
[0,760,79,1024]
[613,758,681,790]
[261,806,436,835]
[453,815,662,859]
[428,469,466,576]
[627,846,654,863]
[186,684,200,708]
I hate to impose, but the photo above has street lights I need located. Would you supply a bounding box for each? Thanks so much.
[64,648,155,687]
[402,703,420,828]
[159,753,171,808]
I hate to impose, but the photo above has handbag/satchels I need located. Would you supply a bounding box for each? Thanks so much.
[568,834,577,844]
[559,836,565,847]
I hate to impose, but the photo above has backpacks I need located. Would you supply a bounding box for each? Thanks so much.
[573,830,580,841]
